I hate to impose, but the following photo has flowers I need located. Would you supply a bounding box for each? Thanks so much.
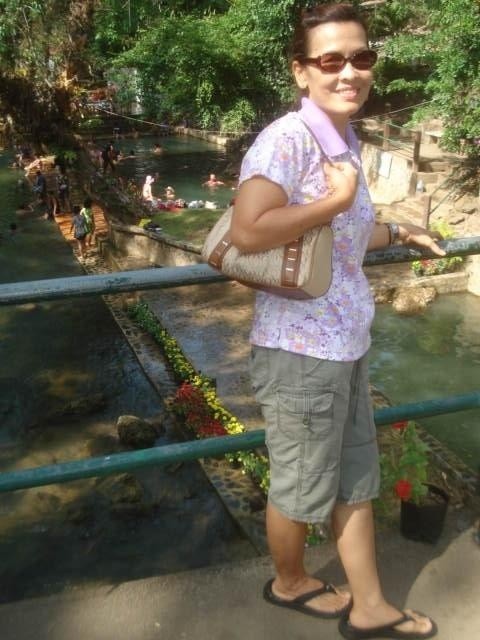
[383,421,432,506]
[409,216,463,278]
[166,373,273,495]
[303,521,323,547]
[128,300,199,385]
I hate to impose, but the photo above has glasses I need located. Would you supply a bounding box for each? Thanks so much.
[303,50,379,75]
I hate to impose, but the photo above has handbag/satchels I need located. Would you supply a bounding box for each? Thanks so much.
[204,203,334,301]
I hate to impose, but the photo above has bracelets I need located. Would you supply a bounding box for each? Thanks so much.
[384,213,401,249]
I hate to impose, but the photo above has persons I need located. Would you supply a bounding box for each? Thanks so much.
[0,112,249,260]
[230,1,447,639]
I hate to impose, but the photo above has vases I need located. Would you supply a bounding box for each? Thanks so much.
[400,484,449,546]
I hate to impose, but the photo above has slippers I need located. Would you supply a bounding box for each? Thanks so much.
[340,607,438,639]
[262,580,349,618]
